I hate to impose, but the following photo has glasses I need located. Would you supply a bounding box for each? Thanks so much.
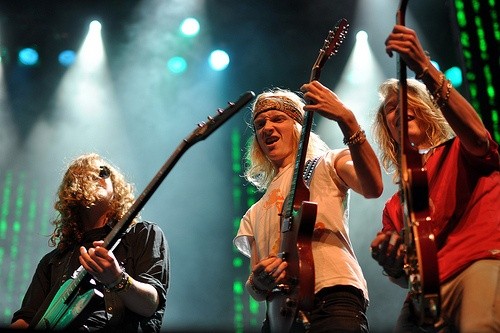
[98,166,110,179]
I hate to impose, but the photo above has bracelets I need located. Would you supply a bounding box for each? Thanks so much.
[415,56,430,80]
[248,273,265,296]
[100,269,134,295]
[343,130,367,147]
[428,74,452,108]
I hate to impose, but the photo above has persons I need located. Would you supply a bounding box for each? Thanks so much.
[233,81,383,333]
[371,25,500,333]
[11,153,170,333]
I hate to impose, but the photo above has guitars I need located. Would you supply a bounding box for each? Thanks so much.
[394,0,448,333]
[34,91,256,333]
[264,8,355,333]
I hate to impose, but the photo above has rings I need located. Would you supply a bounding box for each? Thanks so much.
[401,33,403,40]
